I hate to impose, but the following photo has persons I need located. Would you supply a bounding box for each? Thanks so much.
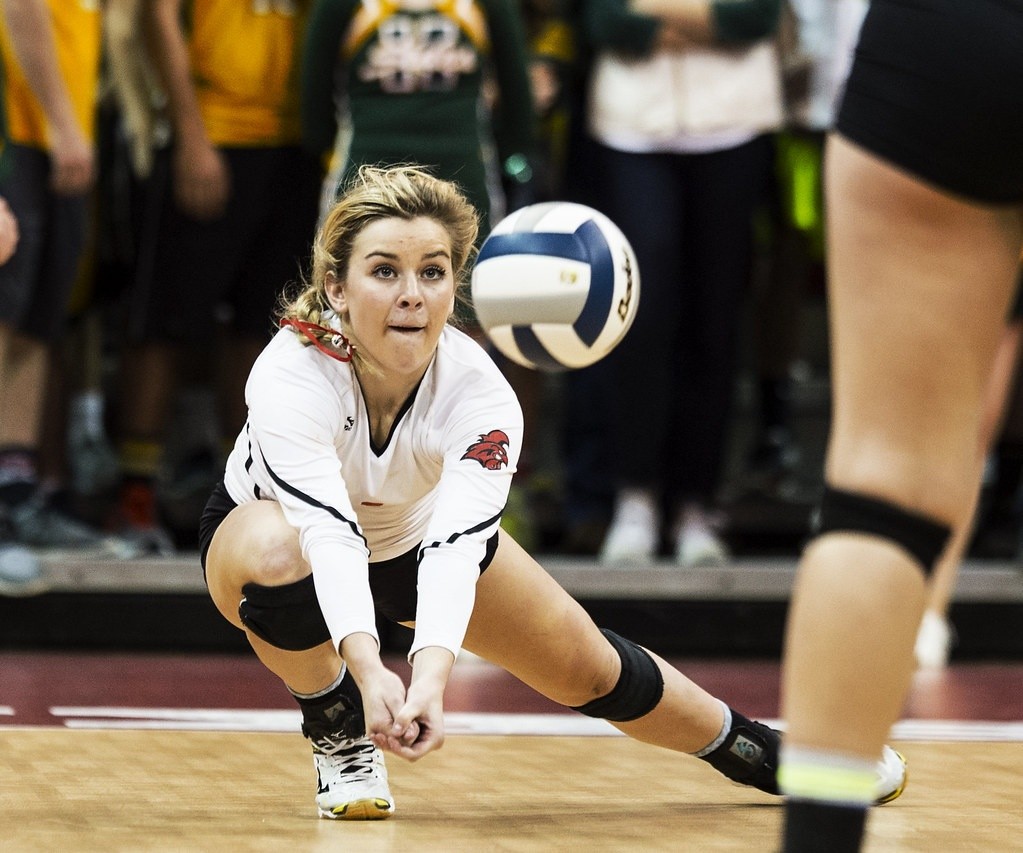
[0,0,1023,853]
[778,1,1023,851]
[199,161,907,820]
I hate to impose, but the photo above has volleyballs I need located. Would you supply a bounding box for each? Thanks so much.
[471,201,641,374]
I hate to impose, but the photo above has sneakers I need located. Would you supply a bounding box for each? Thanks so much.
[872,742,909,804]
[302,706,395,821]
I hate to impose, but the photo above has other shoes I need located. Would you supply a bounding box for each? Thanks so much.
[677,510,726,569]
[600,496,661,568]
[0,471,145,568]
